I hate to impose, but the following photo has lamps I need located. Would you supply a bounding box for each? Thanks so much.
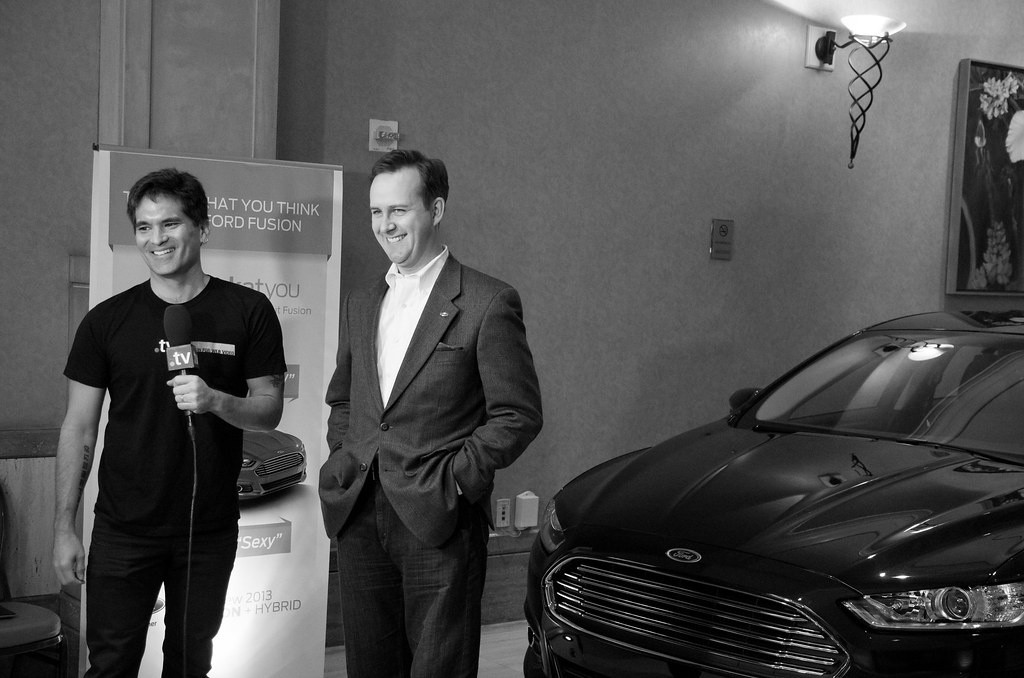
[806,14,907,168]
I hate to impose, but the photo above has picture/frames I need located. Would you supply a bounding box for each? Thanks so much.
[944,59,1024,299]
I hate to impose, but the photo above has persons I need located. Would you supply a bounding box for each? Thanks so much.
[52,168,286,678]
[317,148,544,677]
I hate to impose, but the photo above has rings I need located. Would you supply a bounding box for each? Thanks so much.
[181,394,184,404]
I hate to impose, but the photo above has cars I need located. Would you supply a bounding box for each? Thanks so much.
[235,427,307,501]
[521,308,1024,678]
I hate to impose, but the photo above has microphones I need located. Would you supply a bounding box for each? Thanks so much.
[164,305,196,442]
